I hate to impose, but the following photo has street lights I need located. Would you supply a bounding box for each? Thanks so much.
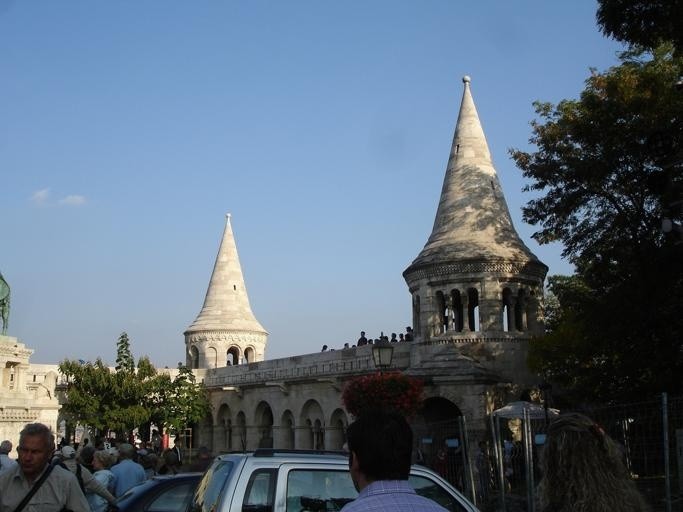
[370,336,394,376]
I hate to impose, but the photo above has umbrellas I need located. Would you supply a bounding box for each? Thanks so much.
[492,401,561,422]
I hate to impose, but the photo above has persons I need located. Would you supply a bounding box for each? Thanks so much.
[339,409,452,512]
[539,411,650,510]
[0,424,212,512]
[424,435,542,511]
[322,326,416,352]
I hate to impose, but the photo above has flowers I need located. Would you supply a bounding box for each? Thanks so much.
[336,368,429,423]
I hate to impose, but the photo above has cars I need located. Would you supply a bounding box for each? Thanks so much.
[90,469,343,512]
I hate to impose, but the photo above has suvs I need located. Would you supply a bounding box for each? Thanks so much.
[186,435,481,512]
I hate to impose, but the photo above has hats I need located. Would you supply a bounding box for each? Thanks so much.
[61,446,76,459]
